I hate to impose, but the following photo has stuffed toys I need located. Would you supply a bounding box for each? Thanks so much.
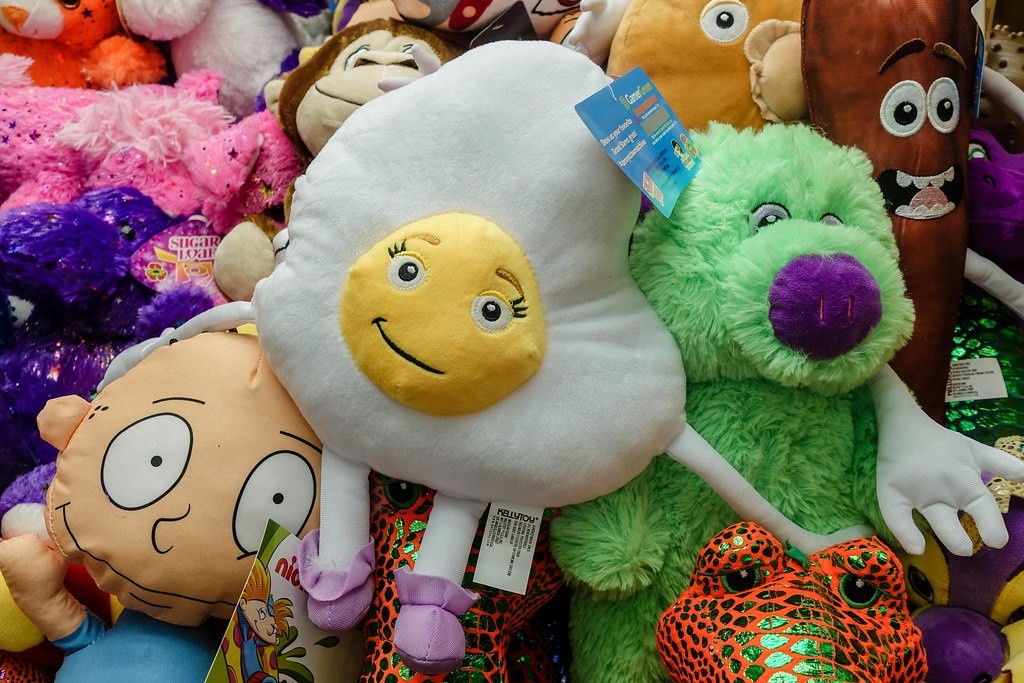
[98,41,878,671]
[0,0,1024,683]
